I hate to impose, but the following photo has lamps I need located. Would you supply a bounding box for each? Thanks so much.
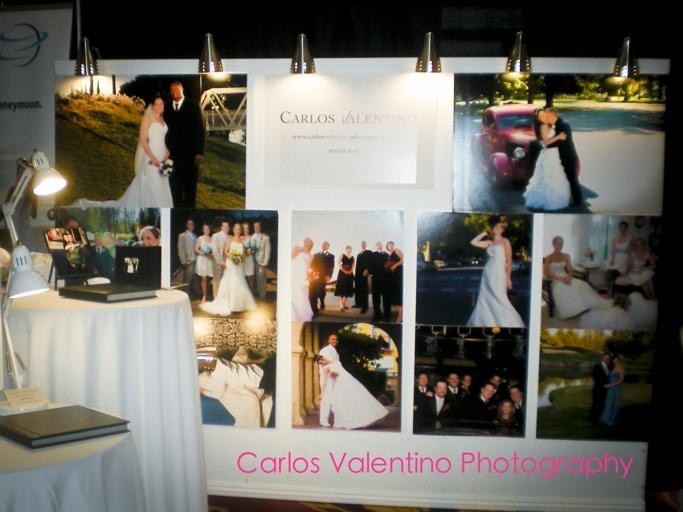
[196,34,221,72]
[291,33,317,75]
[8,148,70,212]
[614,37,637,77]
[504,30,534,75]
[416,31,443,73]
[2,203,51,300]
[75,37,99,78]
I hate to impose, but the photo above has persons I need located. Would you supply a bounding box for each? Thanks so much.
[413,371,433,411]
[489,399,522,439]
[239,222,256,298]
[580,292,658,329]
[197,222,257,316]
[211,218,233,298]
[463,215,526,327]
[522,107,572,211]
[250,220,270,300]
[446,371,467,435]
[371,241,392,323]
[194,221,214,303]
[163,80,206,209]
[101,232,116,275]
[177,217,198,284]
[351,241,373,314]
[461,374,473,393]
[462,382,497,436]
[542,107,583,207]
[605,221,634,275]
[388,241,403,323]
[90,231,108,273]
[64,216,80,228]
[543,236,606,321]
[413,379,453,435]
[60,94,174,208]
[139,226,160,246]
[319,333,339,427]
[315,355,390,430]
[600,353,625,426]
[291,237,314,322]
[309,241,335,319]
[583,351,624,418]
[615,238,656,299]
[127,231,138,247]
[334,245,355,312]
[489,374,504,403]
[509,384,525,425]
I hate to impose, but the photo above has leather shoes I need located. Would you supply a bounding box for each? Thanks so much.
[320,303,325,309]
[373,315,381,321]
[361,305,369,314]
[352,304,359,308]
[385,313,391,321]
[312,311,318,319]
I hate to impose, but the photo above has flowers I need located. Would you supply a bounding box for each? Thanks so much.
[148,157,174,176]
[227,248,242,262]
[201,245,212,254]
[243,237,261,263]
[342,264,359,272]
[384,260,396,273]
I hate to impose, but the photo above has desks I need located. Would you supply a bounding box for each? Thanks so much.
[0,285,209,511]
[1,402,146,512]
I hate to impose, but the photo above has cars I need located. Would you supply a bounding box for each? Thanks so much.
[471,106,580,190]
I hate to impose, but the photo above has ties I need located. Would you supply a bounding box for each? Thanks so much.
[175,103,178,112]
[453,389,456,395]
[422,388,424,393]
[517,403,521,410]
[320,422,331,427]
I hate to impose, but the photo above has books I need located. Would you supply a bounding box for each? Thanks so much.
[0,405,131,450]
[59,282,158,303]
